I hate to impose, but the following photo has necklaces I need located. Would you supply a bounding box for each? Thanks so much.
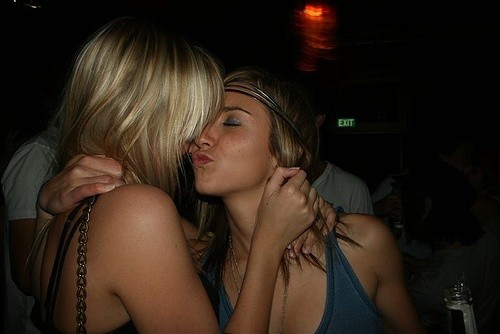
[228,235,289,334]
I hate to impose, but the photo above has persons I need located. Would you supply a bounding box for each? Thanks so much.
[31,67,426,334]
[0,126,77,334]
[307,112,500,334]
[31,15,336,334]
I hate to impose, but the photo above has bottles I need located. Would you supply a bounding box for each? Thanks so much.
[441,281,478,334]
